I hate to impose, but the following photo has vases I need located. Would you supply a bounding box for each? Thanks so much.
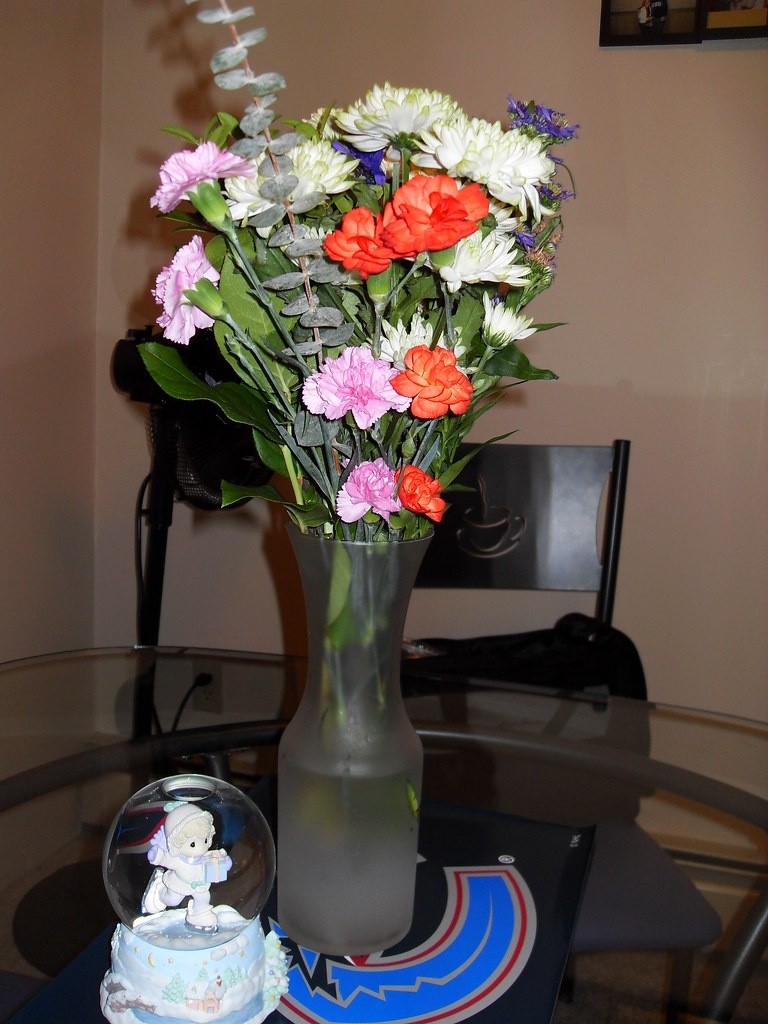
[274,519,438,960]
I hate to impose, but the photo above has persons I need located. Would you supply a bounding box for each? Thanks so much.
[142,800,232,929]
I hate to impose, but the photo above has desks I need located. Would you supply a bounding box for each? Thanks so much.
[0,646,768,1024]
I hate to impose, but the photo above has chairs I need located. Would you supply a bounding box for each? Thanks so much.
[238,427,631,1024]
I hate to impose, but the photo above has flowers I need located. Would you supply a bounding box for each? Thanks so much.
[131,1,584,837]
